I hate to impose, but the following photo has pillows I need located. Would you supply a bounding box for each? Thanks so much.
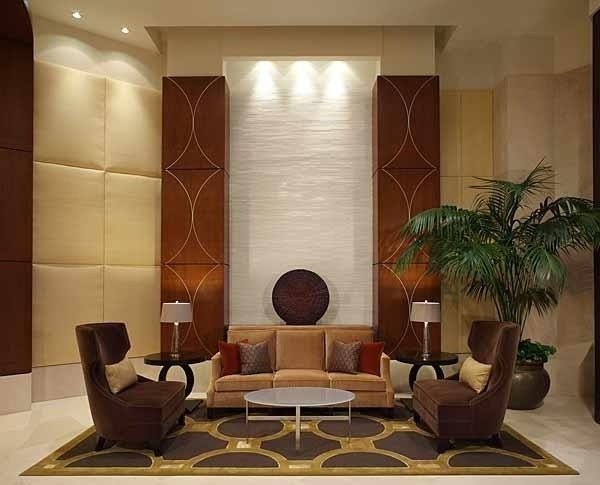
[330,339,358,373]
[354,338,384,377]
[239,339,271,373]
[456,352,493,393]
[105,356,139,397]
[219,339,249,376]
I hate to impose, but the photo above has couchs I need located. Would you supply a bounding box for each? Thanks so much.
[205,326,398,416]
[76,322,190,455]
[408,313,524,451]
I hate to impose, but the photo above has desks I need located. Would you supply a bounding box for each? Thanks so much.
[142,350,208,401]
[397,349,461,394]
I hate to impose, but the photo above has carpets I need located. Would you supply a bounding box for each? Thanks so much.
[19,390,579,479]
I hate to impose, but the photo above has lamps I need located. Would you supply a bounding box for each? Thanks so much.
[410,298,442,359]
[160,301,195,357]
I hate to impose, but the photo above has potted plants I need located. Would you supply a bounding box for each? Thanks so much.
[507,341,555,412]
